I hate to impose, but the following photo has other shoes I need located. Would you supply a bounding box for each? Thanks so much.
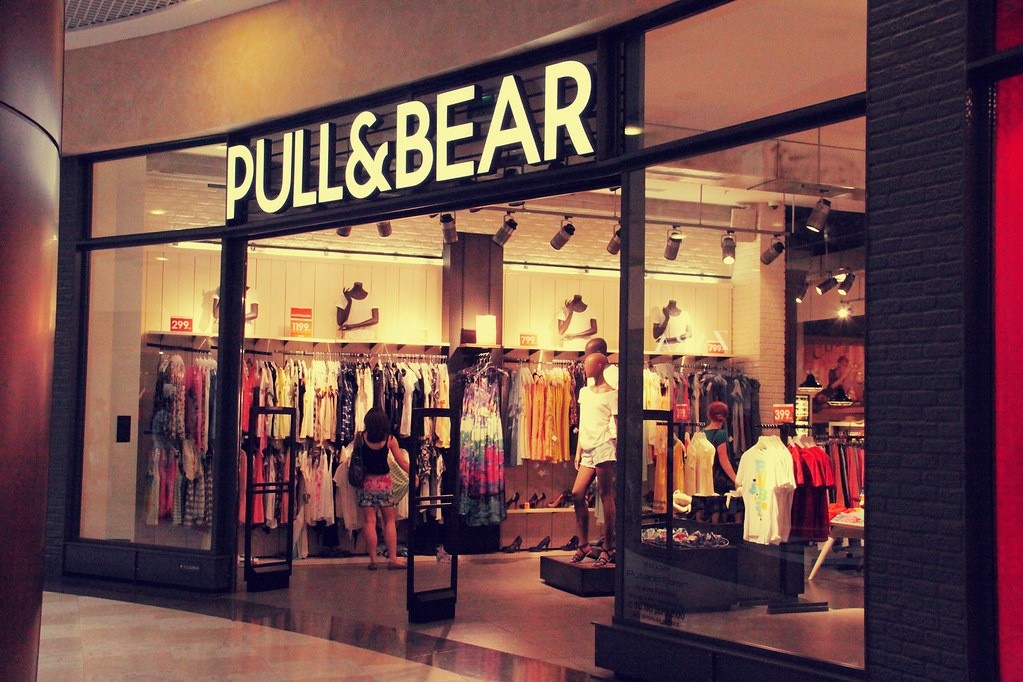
[388,560,407,570]
[368,562,378,570]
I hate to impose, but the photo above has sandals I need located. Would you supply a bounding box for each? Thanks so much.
[594,546,615,566]
[570,543,592,564]
[644,526,729,548]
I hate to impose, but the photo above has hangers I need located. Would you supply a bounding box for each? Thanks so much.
[150,342,448,468]
[454,350,761,391]
[652,419,865,459]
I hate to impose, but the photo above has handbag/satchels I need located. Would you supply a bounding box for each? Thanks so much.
[348,430,364,487]
[712,431,737,493]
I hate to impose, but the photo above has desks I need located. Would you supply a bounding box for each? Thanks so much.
[807,507,865,581]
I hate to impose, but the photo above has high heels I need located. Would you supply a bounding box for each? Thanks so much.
[560,535,579,550]
[531,493,546,509]
[519,492,537,509]
[501,535,522,553]
[685,496,745,524]
[584,492,595,507]
[547,491,567,507]
[506,491,519,509]
[528,536,550,552]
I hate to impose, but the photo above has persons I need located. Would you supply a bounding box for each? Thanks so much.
[354,408,419,569]
[828,356,852,400]
[702,401,737,492]
[571,338,620,567]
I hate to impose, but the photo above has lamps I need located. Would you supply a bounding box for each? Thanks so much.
[549,219,575,250]
[837,270,855,295]
[794,370,823,392]
[663,228,682,261]
[805,191,831,233]
[827,385,853,407]
[816,272,838,297]
[761,237,786,266]
[439,211,458,245]
[795,282,809,303]
[607,222,621,255]
[492,214,518,247]
[337,226,351,237]
[376,220,392,237]
[720,232,737,264]
